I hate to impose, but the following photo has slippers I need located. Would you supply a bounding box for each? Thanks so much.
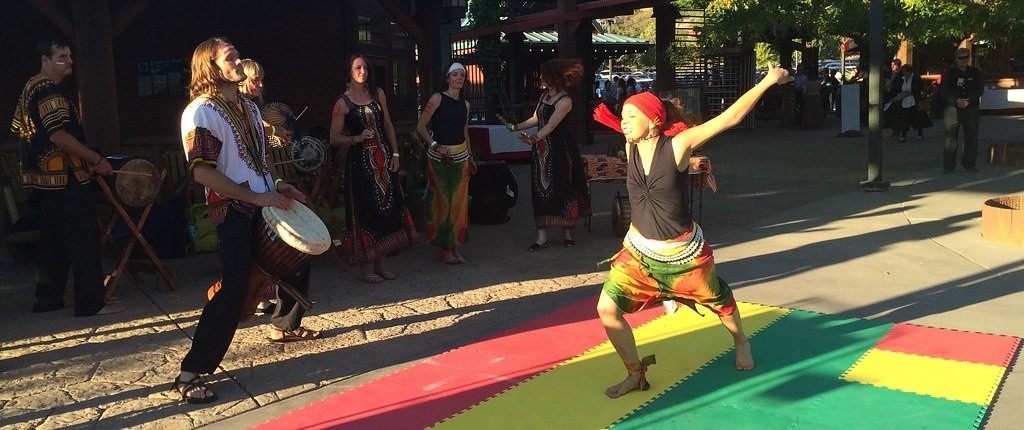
[378,270,398,281]
[254,303,277,315]
[359,272,384,284]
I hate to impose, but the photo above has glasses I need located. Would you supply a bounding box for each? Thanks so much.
[956,56,970,60]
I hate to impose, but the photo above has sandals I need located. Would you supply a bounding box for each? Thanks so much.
[271,326,323,342]
[456,254,467,263]
[170,372,218,404]
[437,254,460,265]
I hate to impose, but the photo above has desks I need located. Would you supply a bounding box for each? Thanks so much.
[584,156,716,232]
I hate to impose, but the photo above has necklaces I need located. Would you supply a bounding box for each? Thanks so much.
[546,93,557,101]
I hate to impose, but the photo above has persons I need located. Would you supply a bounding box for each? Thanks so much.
[415,62,477,263]
[11,38,125,317]
[937,48,984,175]
[594,76,636,100]
[170,37,321,404]
[330,53,420,283]
[883,59,934,142]
[754,63,861,129]
[596,60,796,398]
[504,58,593,253]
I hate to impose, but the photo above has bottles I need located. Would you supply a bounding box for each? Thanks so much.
[187,222,196,238]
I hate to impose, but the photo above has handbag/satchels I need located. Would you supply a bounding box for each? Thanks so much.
[917,100,931,112]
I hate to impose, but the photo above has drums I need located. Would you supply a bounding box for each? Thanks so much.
[612,196,632,238]
[207,195,332,321]
[91,152,162,207]
[288,135,328,174]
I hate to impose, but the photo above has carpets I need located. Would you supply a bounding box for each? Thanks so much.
[243,292,1022,430]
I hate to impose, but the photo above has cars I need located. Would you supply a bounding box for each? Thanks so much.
[594,66,656,101]
[679,66,802,112]
[820,60,860,79]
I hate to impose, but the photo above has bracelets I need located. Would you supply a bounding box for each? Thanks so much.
[512,123,519,131]
[93,157,102,166]
[271,125,276,135]
[393,153,399,157]
[275,178,283,189]
[430,141,440,151]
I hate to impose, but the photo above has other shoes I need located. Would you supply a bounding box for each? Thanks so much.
[916,135,925,142]
[33,294,66,313]
[564,238,575,248]
[896,137,907,143]
[890,133,899,138]
[73,287,108,317]
[963,167,978,173]
[943,163,956,174]
[529,237,549,252]
